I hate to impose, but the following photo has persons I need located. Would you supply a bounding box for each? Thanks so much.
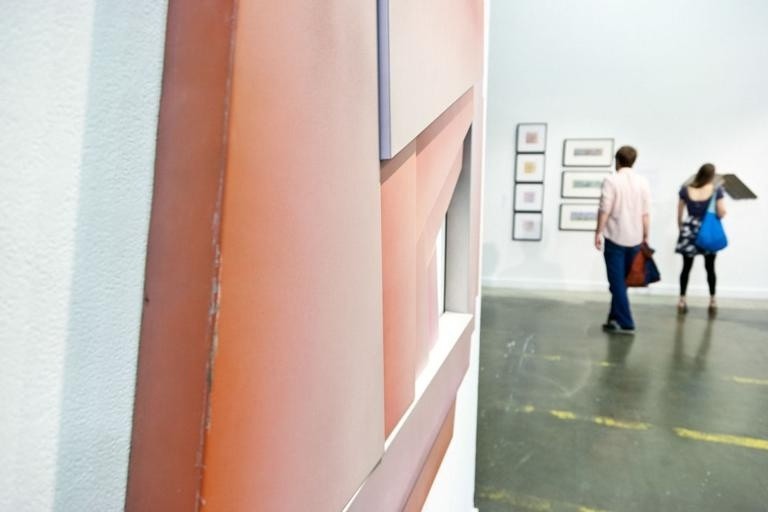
[677,162,726,308]
[593,146,648,334]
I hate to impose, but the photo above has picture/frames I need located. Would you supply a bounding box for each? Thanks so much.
[563,138,615,168]
[561,170,613,199]
[512,122,548,242]
[559,203,601,231]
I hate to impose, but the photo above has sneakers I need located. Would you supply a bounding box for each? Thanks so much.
[603,320,634,333]
[678,305,686,313]
[709,306,717,316]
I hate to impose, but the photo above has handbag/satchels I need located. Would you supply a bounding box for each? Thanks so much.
[696,210,727,251]
[624,244,660,287]
